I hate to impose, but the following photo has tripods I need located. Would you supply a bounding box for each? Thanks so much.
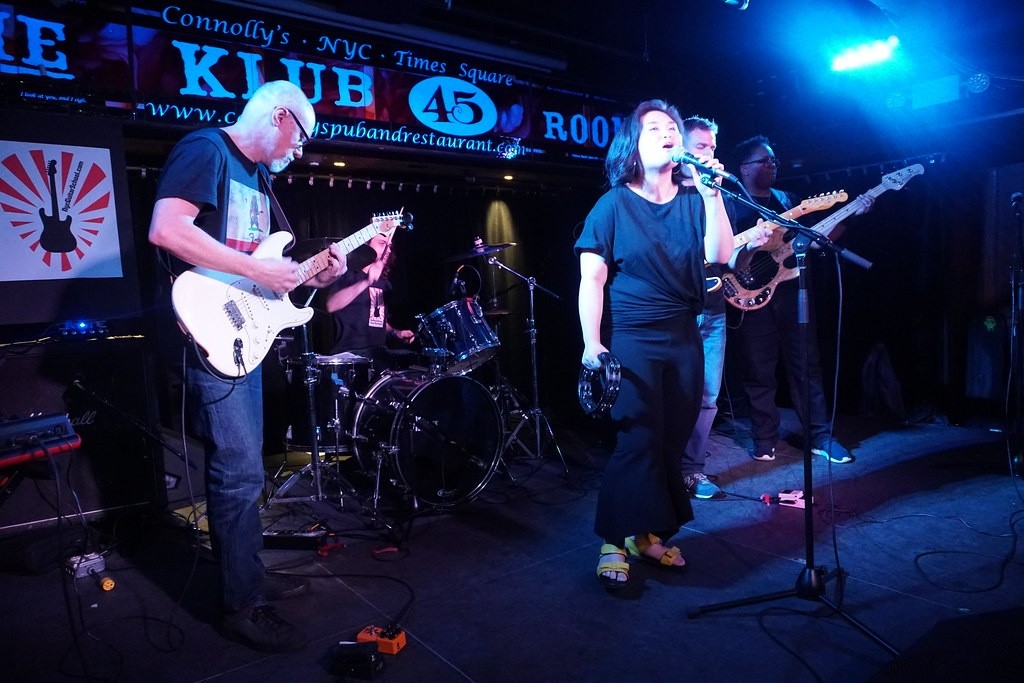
[686,169,901,660]
[484,254,573,492]
[255,323,379,518]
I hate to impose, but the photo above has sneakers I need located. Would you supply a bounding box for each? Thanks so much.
[255,568,310,598]
[811,438,852,463]
[681,472,726,500]
[218,600,310,652]
[752,445,776,460]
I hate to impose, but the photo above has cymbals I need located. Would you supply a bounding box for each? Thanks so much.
[577,352,622,420]
[293,303,330,324]
[484,308,522,321]
[441,242,519,261]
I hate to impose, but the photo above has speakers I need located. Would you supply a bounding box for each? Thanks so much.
[0,333,169,538]
[866,605,1024,683]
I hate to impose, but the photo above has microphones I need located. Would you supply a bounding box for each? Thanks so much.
[670,145,739,183]
[450,272,459,295]
[467,456,488,473]
[73,372,86,385]
[1012,191,1022,210]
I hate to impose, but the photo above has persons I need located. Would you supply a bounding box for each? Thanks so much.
[147,79,348,651]
[673,114,773,501]
[570,97,733,591]
[321,232,422,372]
[719,134,854,465]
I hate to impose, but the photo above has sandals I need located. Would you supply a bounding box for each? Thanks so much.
[597,541,630,585]
[623,531,687,572]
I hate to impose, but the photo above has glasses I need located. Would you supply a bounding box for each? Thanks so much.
[287,110,314,147]
[741,157,780,169]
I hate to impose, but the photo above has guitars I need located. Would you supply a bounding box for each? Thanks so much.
[722,163,924,311]
[703,190,849,294]
[171,210,414,380]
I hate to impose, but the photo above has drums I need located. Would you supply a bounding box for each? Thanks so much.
[416,298,502,377]
[349,368,504,509]
[282,356,375,452]
